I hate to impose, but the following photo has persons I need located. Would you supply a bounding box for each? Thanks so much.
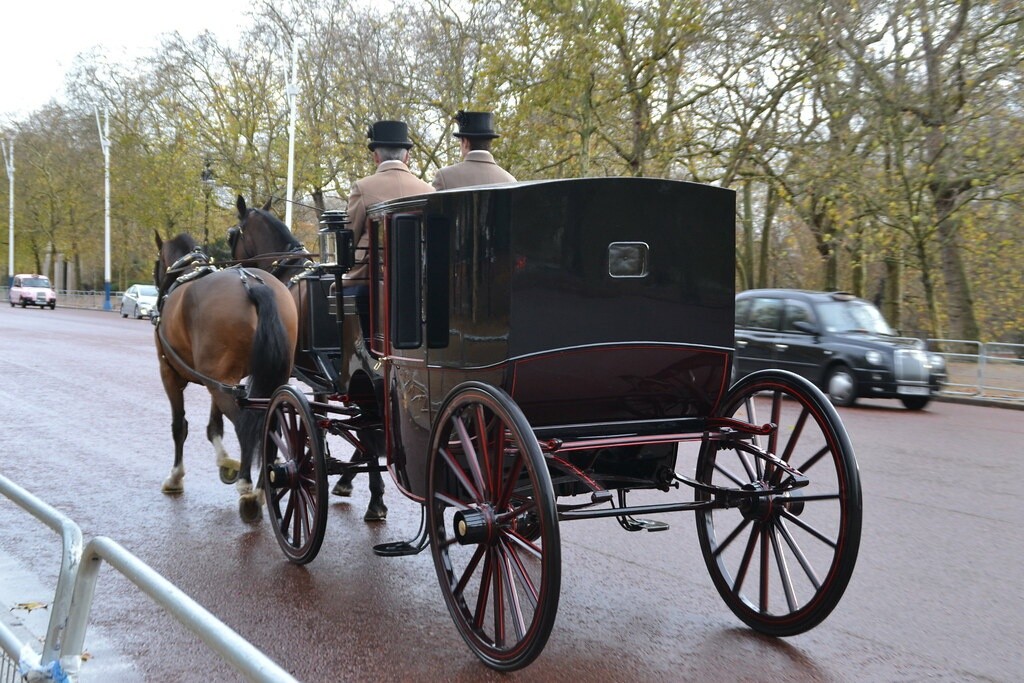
[431,110,517,190]
[344,122,442,359]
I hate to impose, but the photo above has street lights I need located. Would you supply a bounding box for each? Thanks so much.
[1,139,15,290]
[275,32,300,232]
[91,102,115,310]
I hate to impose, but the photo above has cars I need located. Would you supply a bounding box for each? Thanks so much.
[9,273,57,310]
[733,287,950,411]
[120,283,160,319]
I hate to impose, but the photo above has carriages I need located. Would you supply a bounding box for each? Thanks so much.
[148,178,862,674]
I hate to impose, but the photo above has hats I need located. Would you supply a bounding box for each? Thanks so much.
[367,120,414,152]
[453,110,501,138]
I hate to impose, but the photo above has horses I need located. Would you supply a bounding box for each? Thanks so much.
[150,194,388,525]
